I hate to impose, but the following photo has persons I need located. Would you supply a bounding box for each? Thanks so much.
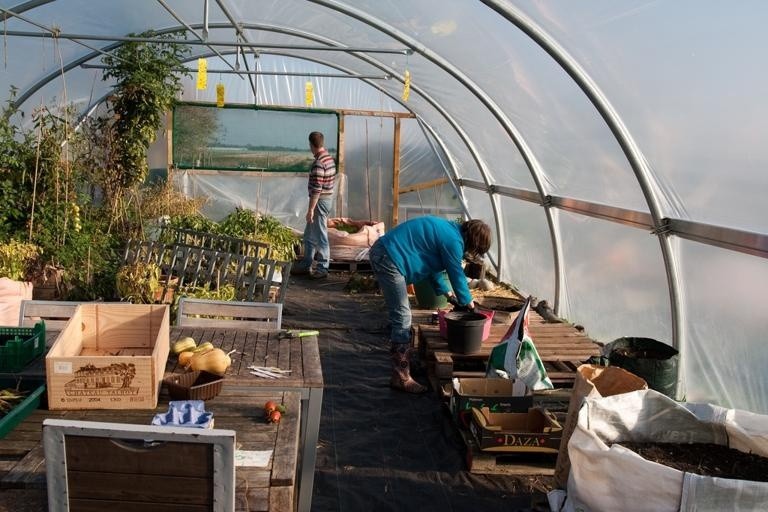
[366,212,493,396]
[288,130,338,281]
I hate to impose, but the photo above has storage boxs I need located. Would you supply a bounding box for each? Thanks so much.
[449,376,564,453]
[45,301,172,411]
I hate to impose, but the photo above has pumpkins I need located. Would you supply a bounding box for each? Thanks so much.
[174,336,231,375]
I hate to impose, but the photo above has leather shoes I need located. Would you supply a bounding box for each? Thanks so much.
[390,374,427,393]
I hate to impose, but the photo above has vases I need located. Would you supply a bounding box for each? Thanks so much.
[27,268,61,299]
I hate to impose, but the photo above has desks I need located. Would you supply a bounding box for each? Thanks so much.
[1,325,324,512]
[0,389,302,510]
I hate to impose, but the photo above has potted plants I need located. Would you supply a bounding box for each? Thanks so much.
[116,261,180,305]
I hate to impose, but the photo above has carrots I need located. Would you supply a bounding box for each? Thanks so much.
[265,400,281,422]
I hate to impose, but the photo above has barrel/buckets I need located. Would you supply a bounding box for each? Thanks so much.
[413,271,454,307]
[444,311,487,353]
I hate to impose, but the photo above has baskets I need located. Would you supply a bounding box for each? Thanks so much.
[0,321,48,372]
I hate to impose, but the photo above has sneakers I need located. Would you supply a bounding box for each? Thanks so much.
[309,271,328,279]
[291,268,312,275]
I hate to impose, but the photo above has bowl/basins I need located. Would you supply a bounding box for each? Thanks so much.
[437,306,495,342]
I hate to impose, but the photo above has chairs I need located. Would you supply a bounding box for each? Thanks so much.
[18,298,284,333]
[38,414,240,512]
